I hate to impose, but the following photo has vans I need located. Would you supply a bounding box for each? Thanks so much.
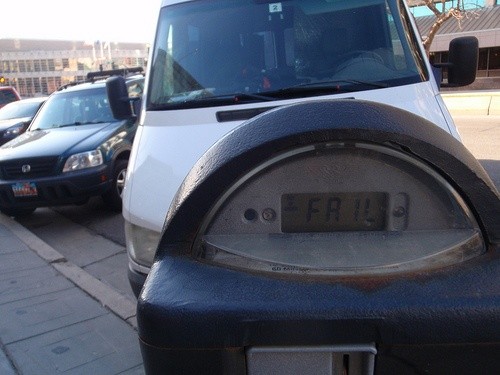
[106,1,500,302]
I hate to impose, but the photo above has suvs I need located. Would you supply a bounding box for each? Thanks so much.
[0,68,145,220]
[0,85,22,107]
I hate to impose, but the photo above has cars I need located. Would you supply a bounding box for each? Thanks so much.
[0,95,53,144]
